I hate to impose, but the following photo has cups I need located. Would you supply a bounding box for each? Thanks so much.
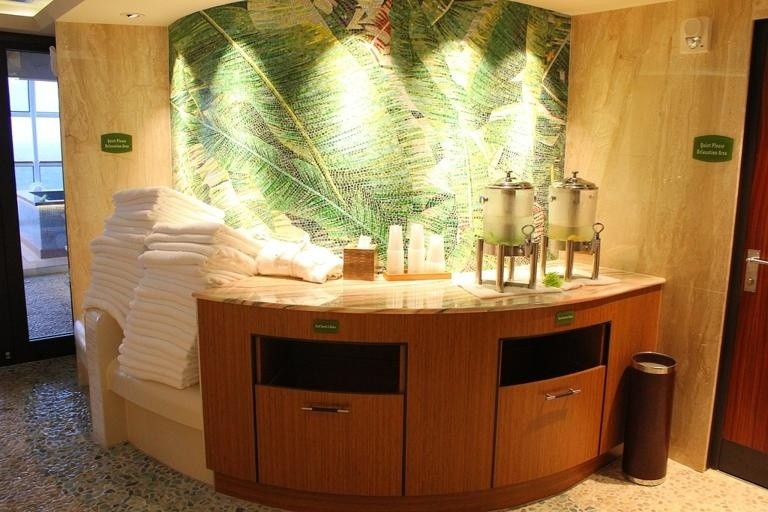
[386,223,404,275]
[425,236,446,274]
[408,225,425,274]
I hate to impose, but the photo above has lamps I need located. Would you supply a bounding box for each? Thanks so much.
[678,18,714,55]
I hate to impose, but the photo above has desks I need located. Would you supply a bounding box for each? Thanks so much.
[197,261,668,505]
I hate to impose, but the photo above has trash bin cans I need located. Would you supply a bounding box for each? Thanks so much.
[624,348,677,488]
